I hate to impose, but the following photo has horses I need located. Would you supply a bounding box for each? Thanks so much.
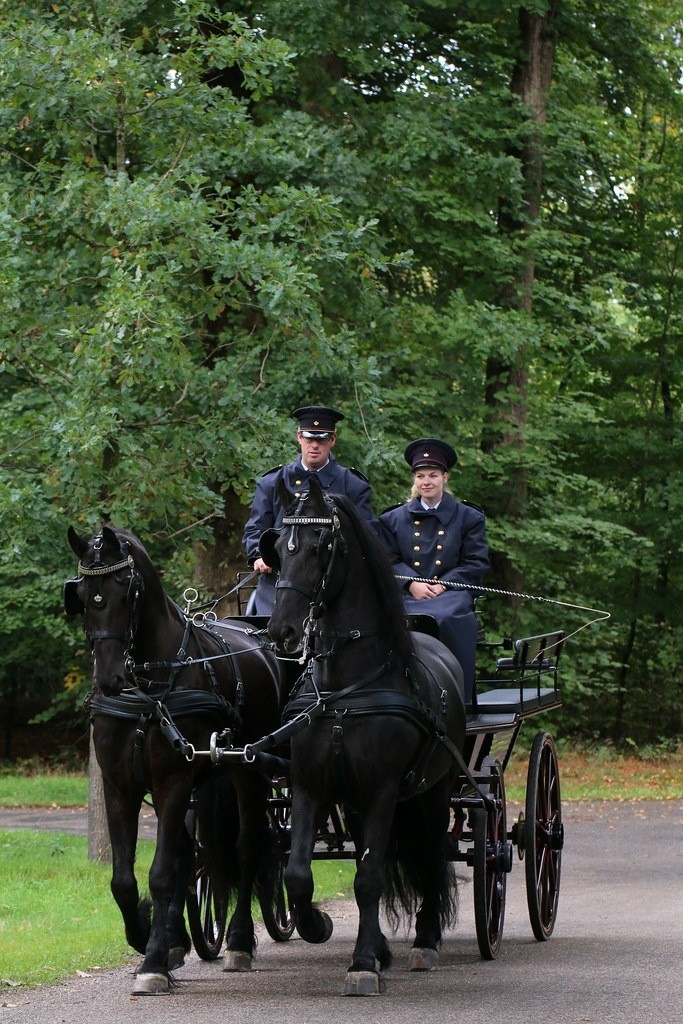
[259,468,468,997]
[63,478,310,996]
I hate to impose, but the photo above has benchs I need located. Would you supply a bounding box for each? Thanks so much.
[234,572,486,717]
[464,630,566,713]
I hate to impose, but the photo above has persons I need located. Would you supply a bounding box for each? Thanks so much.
[378,438,490,707]
[243,406,375,615]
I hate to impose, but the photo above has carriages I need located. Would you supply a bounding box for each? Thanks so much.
[60,473,568,996]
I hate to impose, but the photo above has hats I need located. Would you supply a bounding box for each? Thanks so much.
[293,406,345,439]
[405,439,456,473]
